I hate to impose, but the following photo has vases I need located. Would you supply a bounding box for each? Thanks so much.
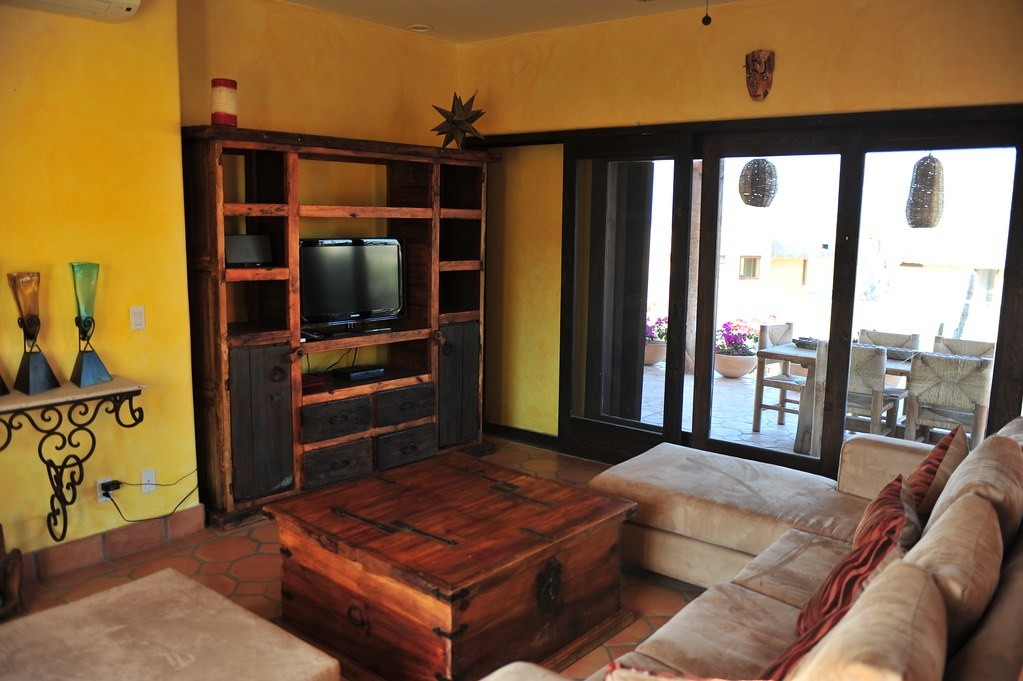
[644,341,668,366]
[714,354,757,378]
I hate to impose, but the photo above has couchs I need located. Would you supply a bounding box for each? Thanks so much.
[477,417,1023,681]
[0,569,341,681]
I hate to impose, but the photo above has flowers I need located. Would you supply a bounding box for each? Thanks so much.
[644,316,668,345]
[713,318,758,357]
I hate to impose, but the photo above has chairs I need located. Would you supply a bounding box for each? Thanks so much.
[748,323,995,451]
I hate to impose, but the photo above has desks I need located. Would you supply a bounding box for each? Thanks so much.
[758,343,912,457]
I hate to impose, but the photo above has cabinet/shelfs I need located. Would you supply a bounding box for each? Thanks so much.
[183,123,501,531]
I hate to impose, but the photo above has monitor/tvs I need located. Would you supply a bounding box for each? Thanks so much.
[299,238,408,338]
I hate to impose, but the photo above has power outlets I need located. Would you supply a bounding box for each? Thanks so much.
[96,479,113,502]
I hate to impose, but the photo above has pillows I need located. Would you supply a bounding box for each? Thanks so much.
[797,427,968,639]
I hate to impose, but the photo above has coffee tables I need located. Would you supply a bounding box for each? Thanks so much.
[262,450,640,681]
